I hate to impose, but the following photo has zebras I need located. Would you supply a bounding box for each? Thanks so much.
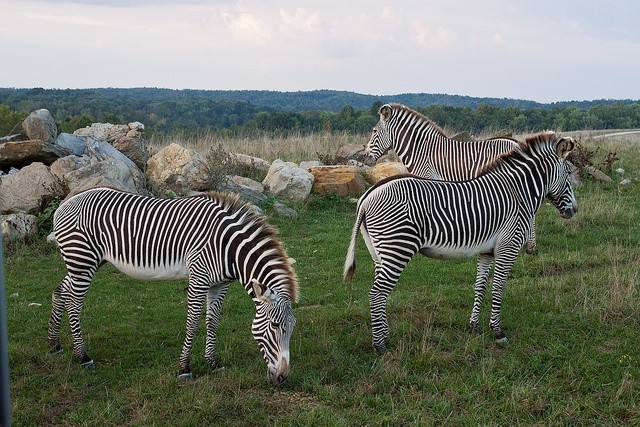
[362,102,538,257]
[342,131,578,356]
[48,185,300,388]
